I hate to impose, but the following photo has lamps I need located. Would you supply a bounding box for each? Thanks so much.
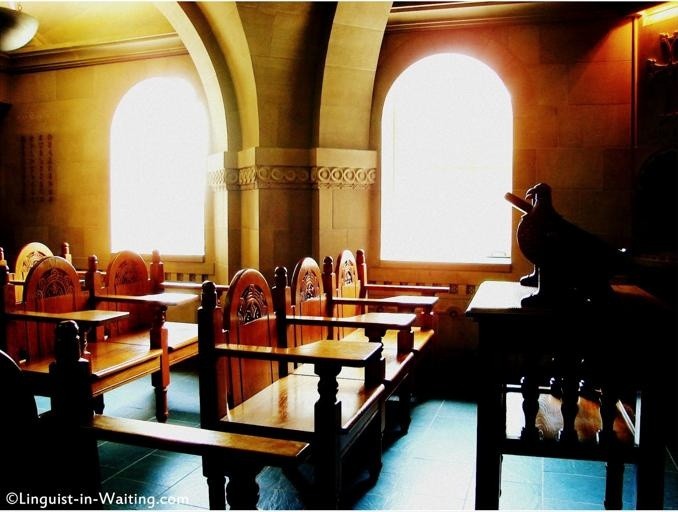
[0,0,40,52]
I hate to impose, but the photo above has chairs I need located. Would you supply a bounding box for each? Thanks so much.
[1,238,451,511]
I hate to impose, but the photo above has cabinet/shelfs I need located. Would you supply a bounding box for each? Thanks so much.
[462,277,666,509]
